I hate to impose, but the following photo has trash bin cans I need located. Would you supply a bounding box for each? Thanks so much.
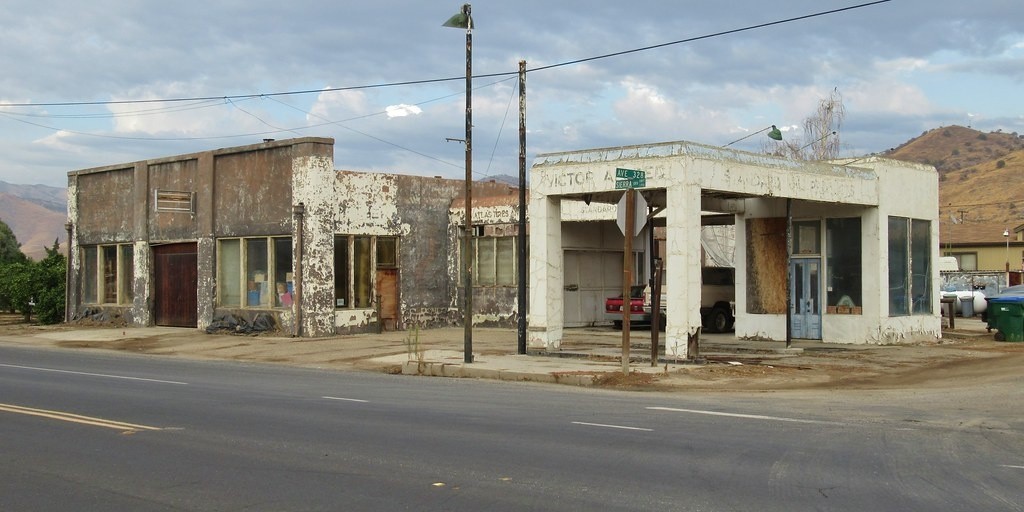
[959,296,975,317]
[984,292,1024,342]
[943,294,957,317]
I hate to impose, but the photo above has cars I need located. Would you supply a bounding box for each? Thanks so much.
[605,267,735,333]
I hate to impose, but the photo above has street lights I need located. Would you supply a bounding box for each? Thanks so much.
[441,2,475,363]
[1003,230,1010,288]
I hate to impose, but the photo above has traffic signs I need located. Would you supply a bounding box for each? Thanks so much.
[615,168,646,188]
